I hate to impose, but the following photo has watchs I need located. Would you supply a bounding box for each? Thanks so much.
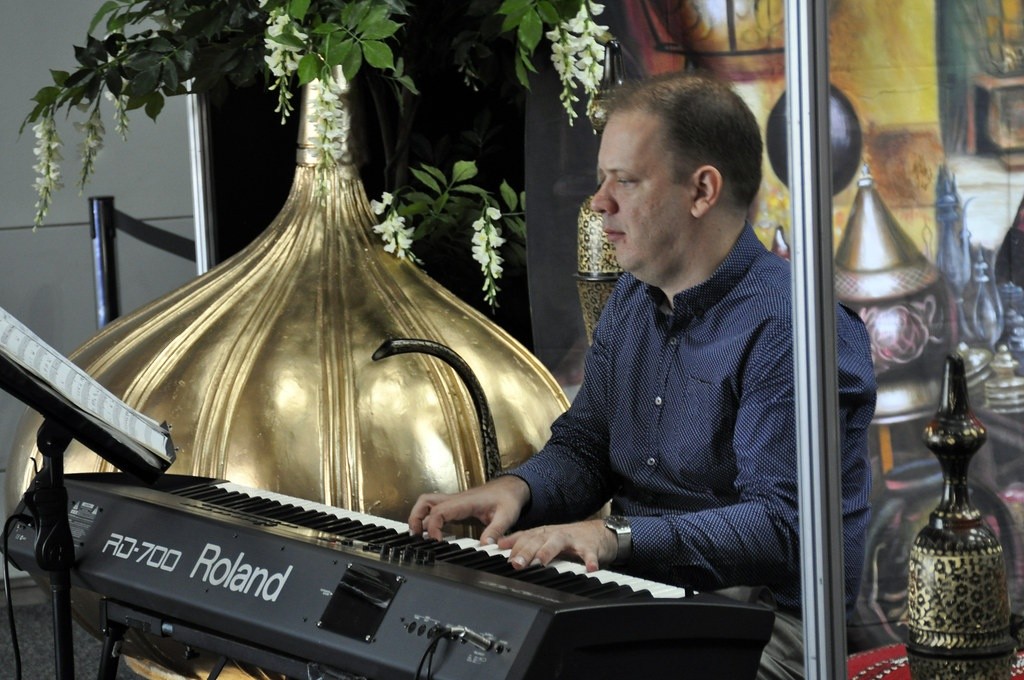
[605,515,632,569]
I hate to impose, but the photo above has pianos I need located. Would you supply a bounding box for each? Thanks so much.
[2,469,777,680]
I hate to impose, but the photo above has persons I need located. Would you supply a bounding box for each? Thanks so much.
[409,77,877,680]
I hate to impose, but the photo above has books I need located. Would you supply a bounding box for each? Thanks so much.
[0,308,173,464]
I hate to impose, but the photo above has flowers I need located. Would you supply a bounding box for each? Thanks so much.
[15,0,618,310]
